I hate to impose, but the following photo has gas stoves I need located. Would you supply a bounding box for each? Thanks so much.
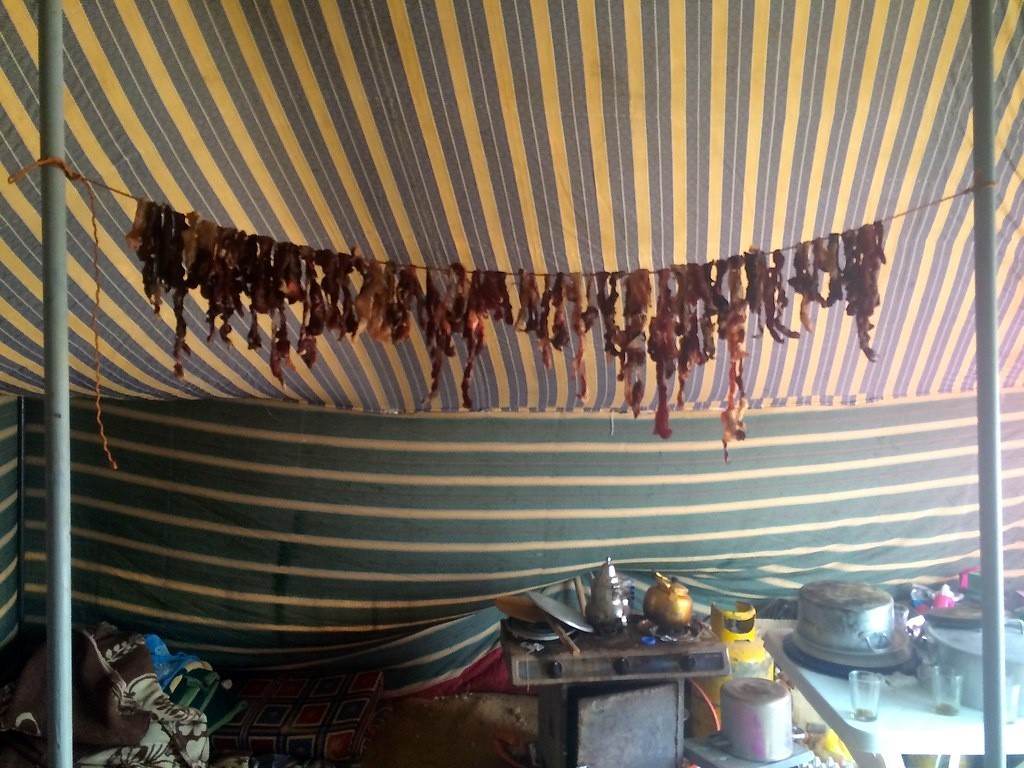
[499,618,729,687]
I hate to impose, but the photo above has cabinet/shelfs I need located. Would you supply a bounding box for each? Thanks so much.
[540,679,685,768]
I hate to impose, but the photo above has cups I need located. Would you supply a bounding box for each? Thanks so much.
[1006,681,1020,725]
[931,666,964,715]
[894,603,909,646]
[848,671,885,721]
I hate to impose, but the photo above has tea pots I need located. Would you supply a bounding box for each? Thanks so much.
[584,556,634,630]
[643,572,693,629]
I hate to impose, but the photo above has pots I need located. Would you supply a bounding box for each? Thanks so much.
[710,677,805,763]
[796,581,894,656]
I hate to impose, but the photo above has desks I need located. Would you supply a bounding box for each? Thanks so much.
[764,627,1024,768]
